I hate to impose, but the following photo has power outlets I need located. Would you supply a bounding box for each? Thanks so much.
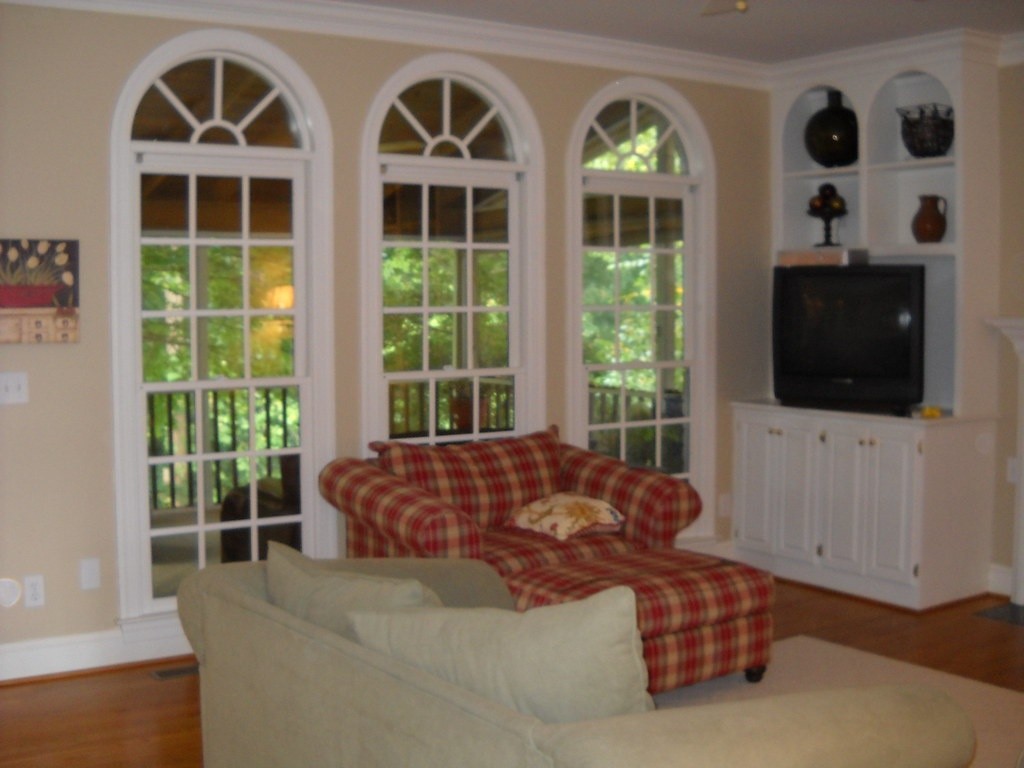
[79,557,102,590]
[22,575,45,608]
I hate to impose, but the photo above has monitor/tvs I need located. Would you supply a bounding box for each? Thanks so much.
[772,262,927,418]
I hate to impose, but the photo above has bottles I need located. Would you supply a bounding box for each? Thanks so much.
[804,91,857,167]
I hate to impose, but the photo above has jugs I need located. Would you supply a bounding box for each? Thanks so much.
[912,195,946,242]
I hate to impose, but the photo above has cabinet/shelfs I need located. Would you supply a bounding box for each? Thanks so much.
[770,24,1002,418]
[728,398,996,613]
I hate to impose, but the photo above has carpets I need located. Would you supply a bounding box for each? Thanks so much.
[652,634,1024,768]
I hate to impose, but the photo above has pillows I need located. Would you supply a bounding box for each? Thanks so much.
[265,540,446,642]
[346,585,658,724]
[503,491,627,543]
[368,423,563,530]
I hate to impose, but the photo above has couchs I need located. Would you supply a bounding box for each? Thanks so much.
[176,555,978,768]
[220,428,516,563]
[317,440,776,695]
